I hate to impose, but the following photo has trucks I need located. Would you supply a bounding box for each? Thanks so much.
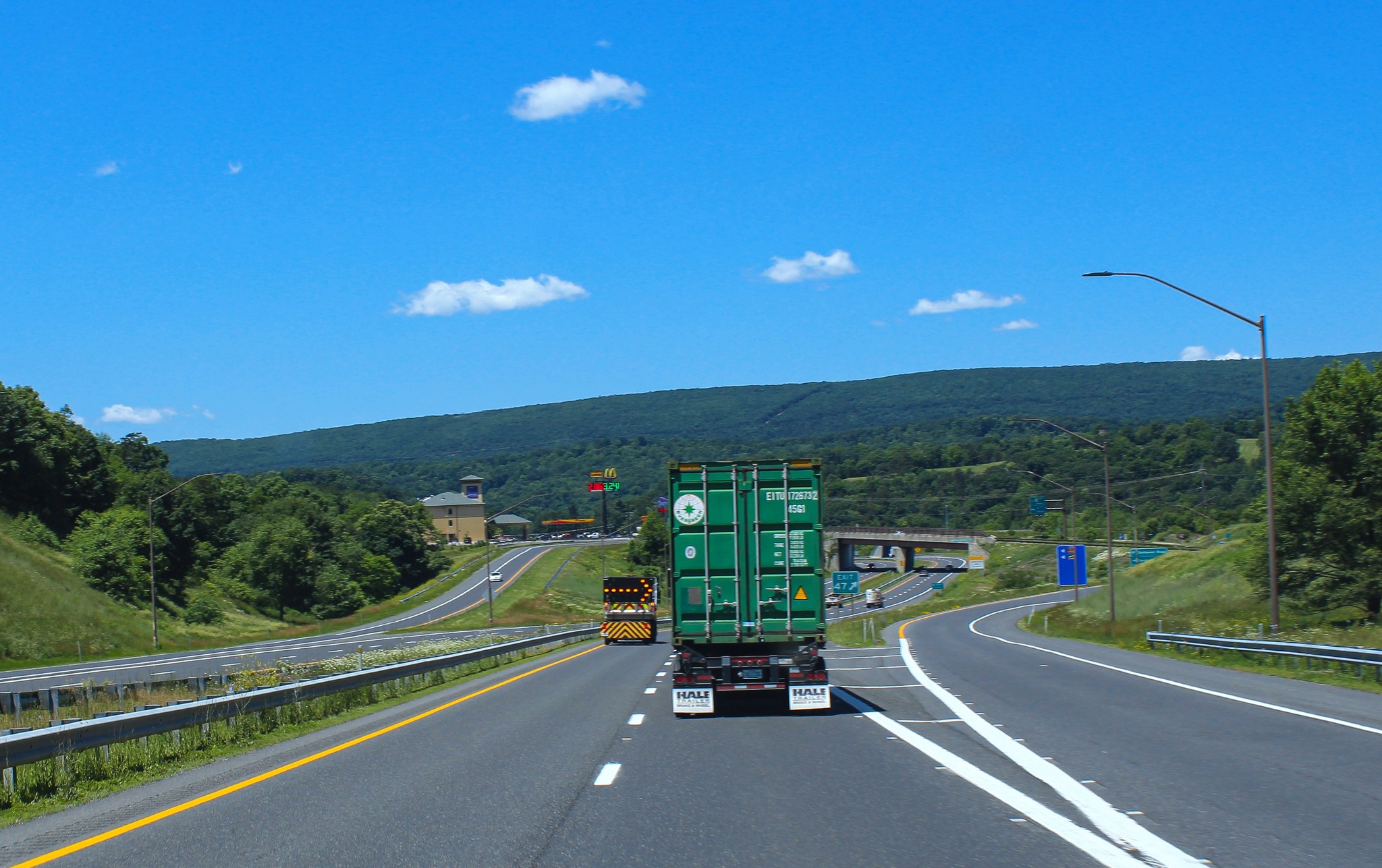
[864,588,885,608]
[666,456,831,720]
[600,573,659,645]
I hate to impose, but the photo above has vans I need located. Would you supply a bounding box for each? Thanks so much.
[464,537,472,544]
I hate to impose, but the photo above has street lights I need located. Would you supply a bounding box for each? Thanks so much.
[1085,492,1139,565]
[483,492,554,624]
[149,471,228,648]
[1081,270,1281,638]
[1006,415,1115,623]
[1192,493,1222,545]
[1171,502,1213,548]
[1005,469,1079,603]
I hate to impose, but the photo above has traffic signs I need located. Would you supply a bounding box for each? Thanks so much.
[832,571,860,594]
[1130,546,1167,566]
[1028,495,1048,514]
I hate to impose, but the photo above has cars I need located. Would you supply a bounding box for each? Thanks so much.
[451,541,457,546]
[489,572,503,582]
[825,595,843,608]
[945,564,954,571]
[531,531,600,540]
[488,535,518,545]
[867,564,876,570]
[919,570,928,577]
